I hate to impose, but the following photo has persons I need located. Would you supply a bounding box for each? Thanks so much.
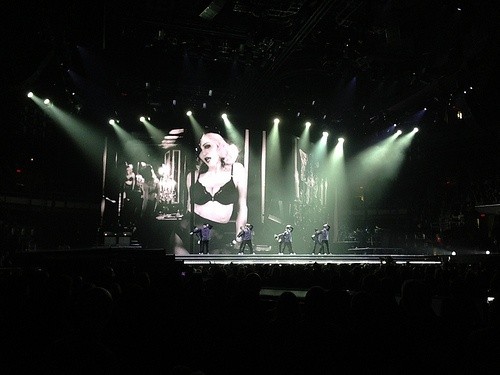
[0,257,500,375]
[137,160,159,187]
[190,222,213,255]
[152,163,180,217]
[273,225,297,255]
[237,222,255,254]
[173,131,249,257]
[143,178,161,217]
[133,173,149,218]
[312,223,332,255]
[124,162,137,202]
